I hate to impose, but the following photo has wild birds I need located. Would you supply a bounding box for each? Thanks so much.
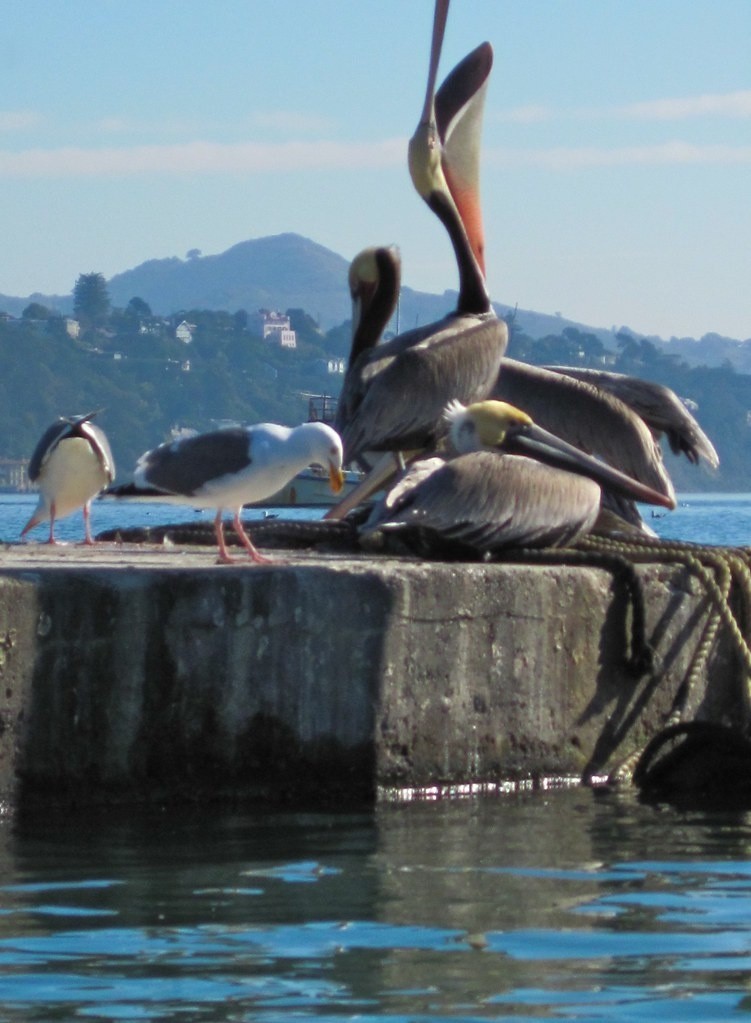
[18,410,117,545]
[134,421,343,565]
[325,0,719,557]
